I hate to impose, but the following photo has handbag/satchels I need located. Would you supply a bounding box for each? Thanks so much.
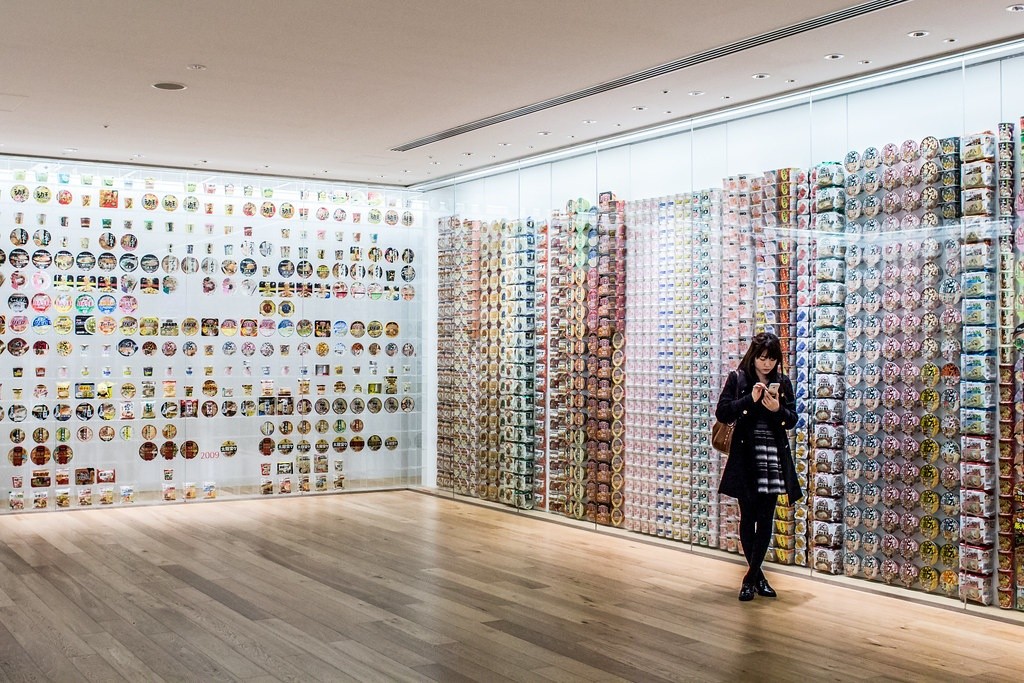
[712,369,742,454]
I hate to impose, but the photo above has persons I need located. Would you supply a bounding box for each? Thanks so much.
[715,331,805,601]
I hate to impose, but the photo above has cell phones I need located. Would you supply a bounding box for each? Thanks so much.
[769,383,780,396]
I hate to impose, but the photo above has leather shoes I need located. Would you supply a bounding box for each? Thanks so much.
[738,580,753,601]
[754,579,776,598]
